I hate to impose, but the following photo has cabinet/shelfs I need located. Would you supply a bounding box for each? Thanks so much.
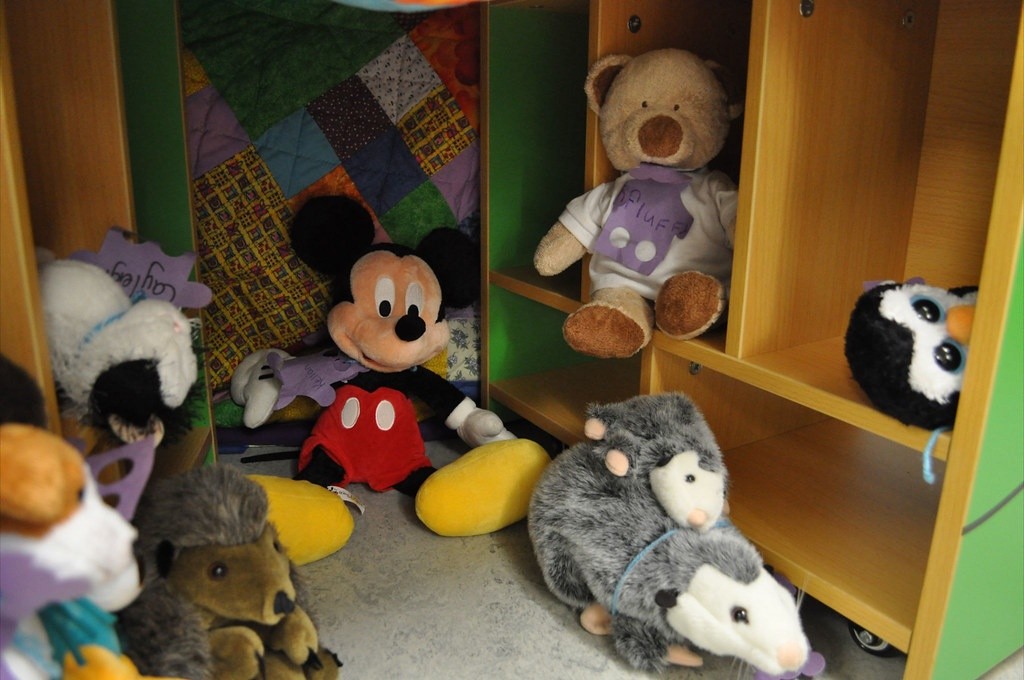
[471,2,1023,679]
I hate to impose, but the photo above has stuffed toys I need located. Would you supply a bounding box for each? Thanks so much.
[533,50,739,358]
[237,197,552,565]
[0,423,182,679]
[842,276,979,487]
[538,499,827,679]
[29,241,199,474]
[528,391,730,609]
[119,463,341,680]
